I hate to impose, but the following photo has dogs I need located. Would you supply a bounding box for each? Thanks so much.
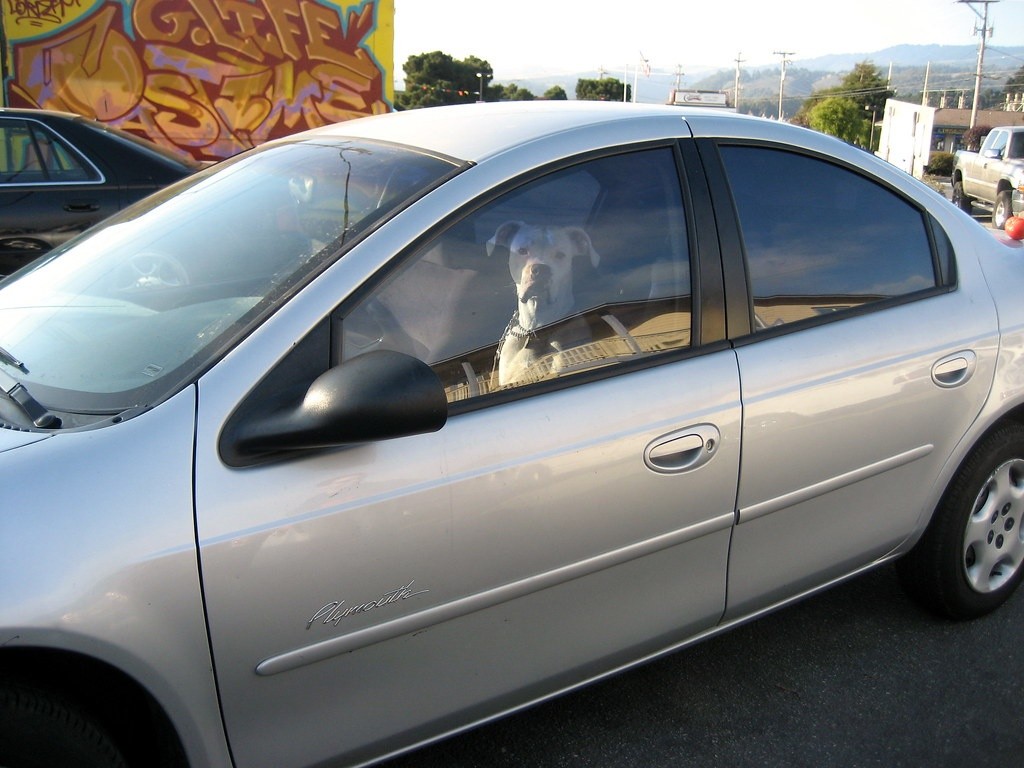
[485,217,601,390]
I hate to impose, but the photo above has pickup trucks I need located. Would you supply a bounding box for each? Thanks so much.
[951,127,1024,228]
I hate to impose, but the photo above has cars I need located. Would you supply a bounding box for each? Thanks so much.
[0,99,1024,768]
[0,107,215,283]
